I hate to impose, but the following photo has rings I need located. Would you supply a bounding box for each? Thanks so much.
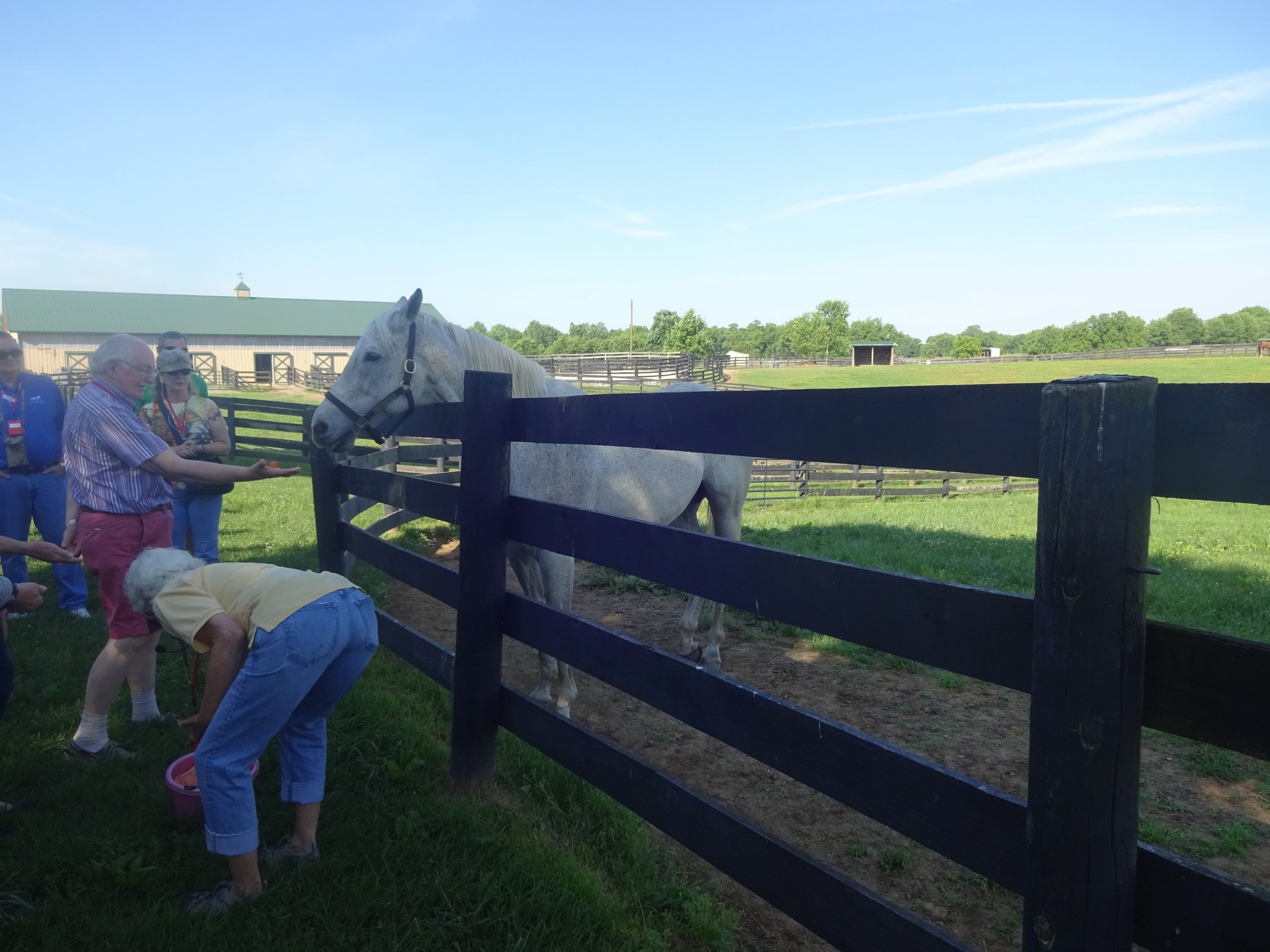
[187,446,189,449]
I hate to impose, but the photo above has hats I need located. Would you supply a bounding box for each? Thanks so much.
[155,347,195,373]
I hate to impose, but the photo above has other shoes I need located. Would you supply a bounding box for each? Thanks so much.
[7,611,35,627]
[71,607,92,619]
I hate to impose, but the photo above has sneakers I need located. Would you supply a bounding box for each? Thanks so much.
[128,714,177,736]
[260,840,319,866]
[190,880,248,911]
[63,734,135,766]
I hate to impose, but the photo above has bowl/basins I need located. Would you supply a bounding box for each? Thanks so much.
[165,751,261,819]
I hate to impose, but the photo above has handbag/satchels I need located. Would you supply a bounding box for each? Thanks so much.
[183,454,234,495]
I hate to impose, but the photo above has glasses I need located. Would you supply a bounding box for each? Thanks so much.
[161,346,188,353]
[163,369,192,376]
[113,358,158,378]
[0,349,23,361]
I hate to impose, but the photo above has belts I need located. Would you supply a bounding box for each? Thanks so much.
[82,502,172,514]
[0,459,60,475]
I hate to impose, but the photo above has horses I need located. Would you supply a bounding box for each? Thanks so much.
[312,288,756,720]
[1258,341,1270,357]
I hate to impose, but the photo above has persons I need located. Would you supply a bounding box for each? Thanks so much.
[61,334,300,763]
[0,573,47,723]
[136,331,230,562]
[126,546,379,916]
[0,536,83,566]
[0,331,93,623]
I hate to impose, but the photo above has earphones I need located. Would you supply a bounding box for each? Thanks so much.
[189,378,191,381]
[160,377,163,382]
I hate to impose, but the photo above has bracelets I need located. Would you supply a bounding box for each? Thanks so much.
[196,444,205,457]
[1,581,20,608]
[66,518,78,528]
